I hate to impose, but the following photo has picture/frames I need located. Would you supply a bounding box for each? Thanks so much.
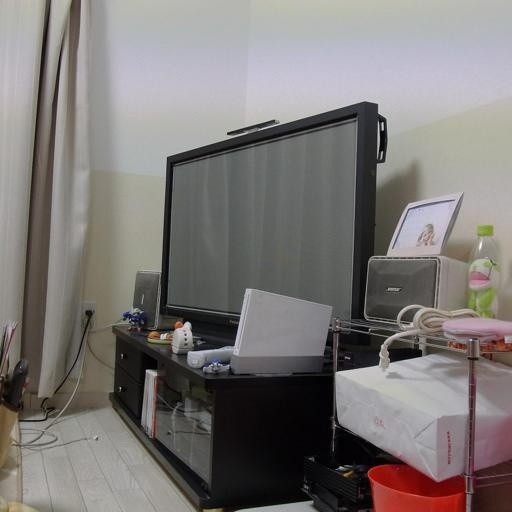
[386,191,465,256]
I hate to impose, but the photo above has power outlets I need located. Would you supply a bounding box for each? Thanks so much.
[80,301,95,332]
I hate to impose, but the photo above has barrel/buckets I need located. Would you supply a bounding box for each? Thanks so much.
[367,465,466,511]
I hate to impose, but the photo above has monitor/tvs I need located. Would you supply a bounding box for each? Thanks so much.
[158,100,388,344]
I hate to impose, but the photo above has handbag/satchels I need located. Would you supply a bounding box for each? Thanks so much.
[335,350,512,483]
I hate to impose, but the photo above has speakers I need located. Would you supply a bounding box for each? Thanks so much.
[131,270,162,331]
[362,254,470,326]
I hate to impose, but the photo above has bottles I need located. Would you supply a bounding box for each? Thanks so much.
[467,225,502,318]
